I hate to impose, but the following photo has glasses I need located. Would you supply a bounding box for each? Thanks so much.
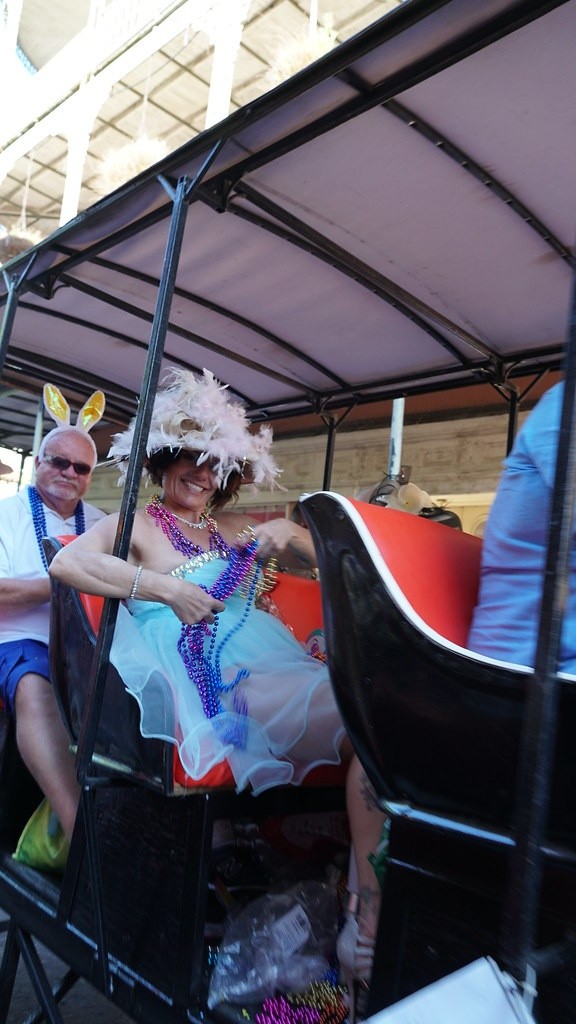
[39,455,94,476]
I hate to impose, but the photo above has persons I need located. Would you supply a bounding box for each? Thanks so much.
[465,368,576,678]
[47,366,391,1023]
[0,426,115,849]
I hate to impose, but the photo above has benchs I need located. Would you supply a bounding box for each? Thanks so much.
[296,483,576,1024]
[45,535,358,1021]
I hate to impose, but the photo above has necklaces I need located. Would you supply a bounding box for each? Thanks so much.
[145,492,232,559]
[168,512,206,531]
[27,486,86,579]
[176,521,281,750]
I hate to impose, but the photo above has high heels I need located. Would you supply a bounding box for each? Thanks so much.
[333,918,396,997]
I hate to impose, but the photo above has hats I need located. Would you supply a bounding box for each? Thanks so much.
[390,481,432,519]
[106,399,272,471]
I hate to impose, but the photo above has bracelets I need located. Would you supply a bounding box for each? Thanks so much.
[129,564,144,602]
